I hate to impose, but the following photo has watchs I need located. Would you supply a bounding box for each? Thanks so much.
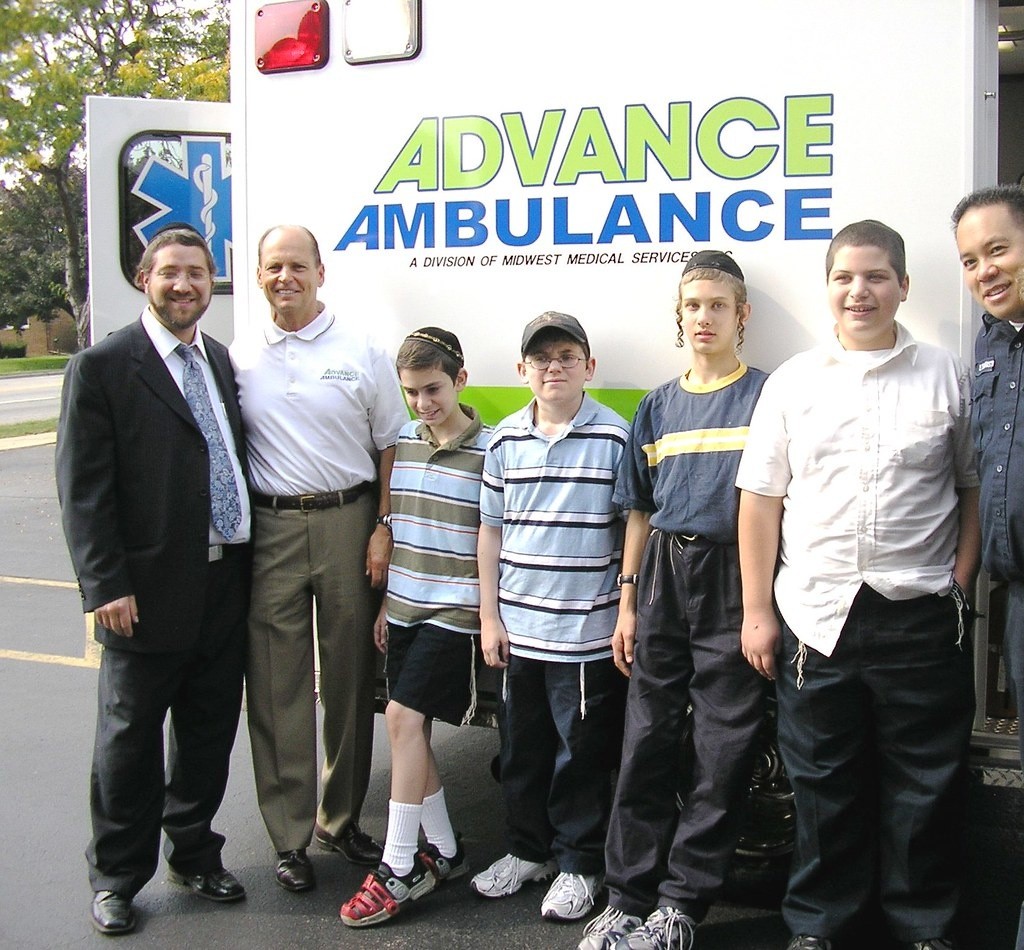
[376,514,392,531]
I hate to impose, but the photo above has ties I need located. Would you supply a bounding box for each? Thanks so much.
[175,344,242,542]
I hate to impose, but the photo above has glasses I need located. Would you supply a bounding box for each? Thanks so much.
[524,353,587,371]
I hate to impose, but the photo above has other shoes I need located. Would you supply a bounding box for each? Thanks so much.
[916,937,963,950]
[787,933,834,950]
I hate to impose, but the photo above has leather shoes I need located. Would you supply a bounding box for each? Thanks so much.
[314,823,384,866]
[89,890,136,934]
[275,848,317,891]
[167,867,248,902]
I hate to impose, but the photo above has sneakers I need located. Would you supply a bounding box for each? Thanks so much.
[470,854,560,899]
[420,838,473,880]
[609,907,699,950]
[575,906,644,950]
[540,872,606,920]
[340,848,437,928]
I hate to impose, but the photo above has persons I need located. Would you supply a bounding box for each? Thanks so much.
[226,223,414,891]
[952,187,1024,767]
[340,324,497,928]
[53,223,256,930]
[736,217,984,950]
[473,310,631,920]
[571,250,776,950]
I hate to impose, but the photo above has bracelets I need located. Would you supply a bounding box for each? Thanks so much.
[616,573,639,587]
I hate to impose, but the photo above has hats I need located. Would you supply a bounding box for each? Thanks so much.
[521,311,591,358]
[405,327,464,367]
[682,249,746,281]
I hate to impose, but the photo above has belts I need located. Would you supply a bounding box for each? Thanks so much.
[209,544,253,562]
[251,481,370,513]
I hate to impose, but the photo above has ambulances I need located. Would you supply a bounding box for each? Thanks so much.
[83,0,1023,911]
